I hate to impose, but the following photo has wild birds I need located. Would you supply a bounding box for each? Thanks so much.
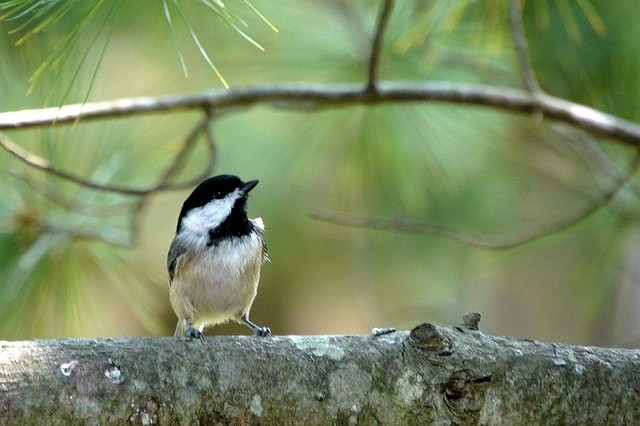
[167,174,272,342]
